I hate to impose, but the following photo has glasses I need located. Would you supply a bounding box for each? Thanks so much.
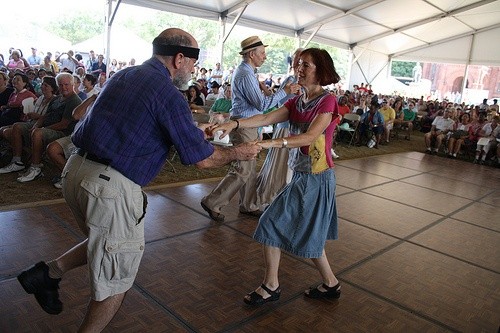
[383,101,387,104]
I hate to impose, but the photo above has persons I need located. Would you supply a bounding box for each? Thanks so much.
[0,54,6,71]
[207,47,341,305]
[474,116,500,164]
[47,92,100,188]
[186,63,232,144]
[87,51,106,76]
[201,36,302,221]
[40,56,56,76]
[17,28,262,333]
[256,48,304,212]
[0,71,39,165]
[329,90,428,148]
[0,72,83,182]
[55,51,85,75]
[419,99,500,133]
[1,48,30,72]
[447,113,474,154]
[424,108,456,155]
[59,66,115,101]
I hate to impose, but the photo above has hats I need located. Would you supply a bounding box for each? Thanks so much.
[239,36,269,55]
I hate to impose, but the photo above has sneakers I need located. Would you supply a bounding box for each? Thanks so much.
[52,182,63,189]
[0,162,26,174]
[16,166,45,183]
[17,261,64,315]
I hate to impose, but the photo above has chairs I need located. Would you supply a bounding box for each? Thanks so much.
[333,113,360,148]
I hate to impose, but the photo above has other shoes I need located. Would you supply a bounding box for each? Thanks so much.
[239,204,265,217]
[201,198,225,221]
[332,153,340,159]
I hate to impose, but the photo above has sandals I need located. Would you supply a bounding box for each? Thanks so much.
[304,280,342,299]
[242,282,281,306]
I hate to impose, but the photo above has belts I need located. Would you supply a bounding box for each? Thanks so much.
[78,147,113,166]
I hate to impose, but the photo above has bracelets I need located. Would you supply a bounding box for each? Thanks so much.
[281,138,287,148]
[233,119,239,129]
